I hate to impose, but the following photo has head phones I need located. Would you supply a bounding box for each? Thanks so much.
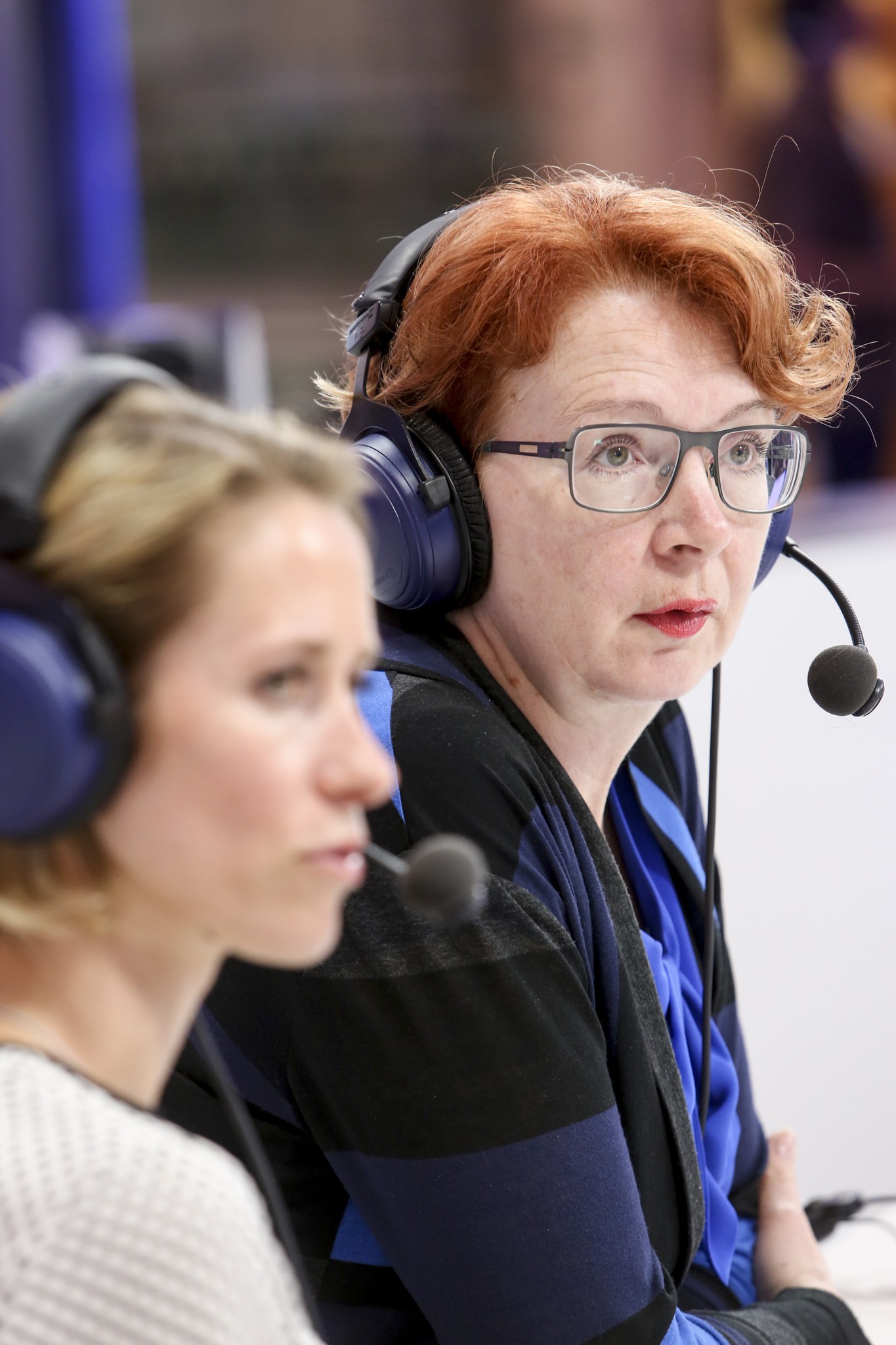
[336,197,794,616]
[0,352,192,846]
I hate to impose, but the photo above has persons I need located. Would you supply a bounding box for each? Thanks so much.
[157,170,875,1345]
[0,353,400,1345]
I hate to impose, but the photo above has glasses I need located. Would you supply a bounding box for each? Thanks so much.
[482,424,810,512]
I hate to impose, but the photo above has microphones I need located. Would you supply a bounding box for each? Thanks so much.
[790,549,885,717]
[358,837,486,924]
